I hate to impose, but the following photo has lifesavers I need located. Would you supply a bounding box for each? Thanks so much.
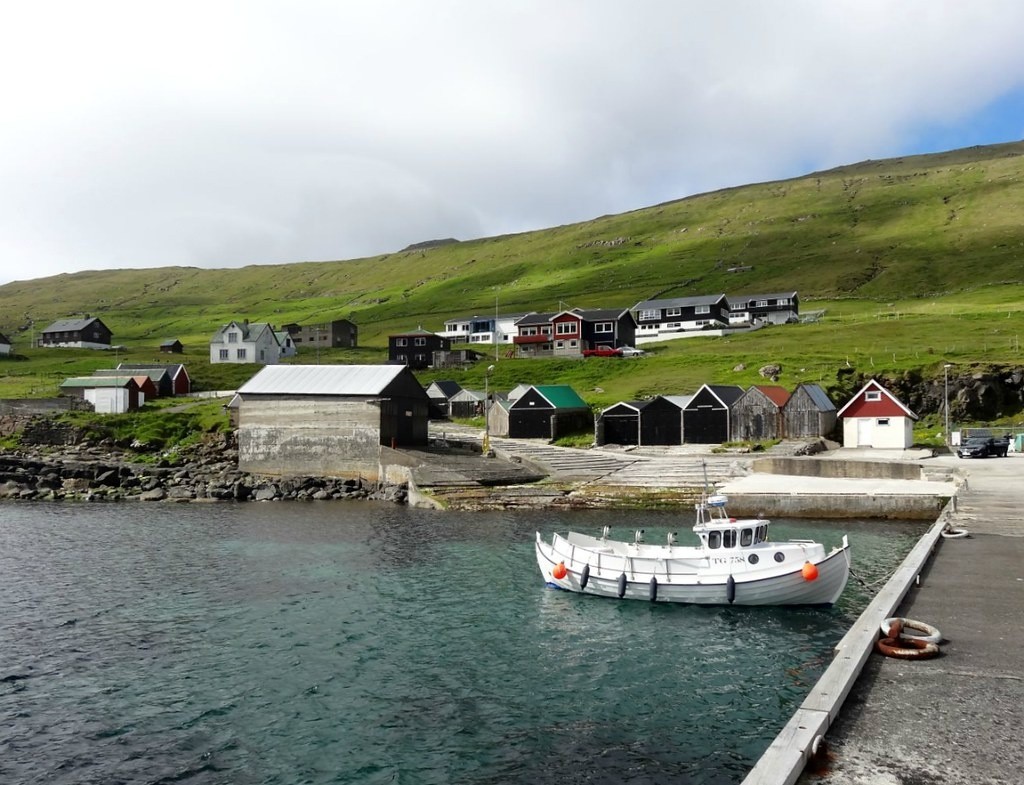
[941,529,969,538]
[881,618,941,643]
[878,637,941,658]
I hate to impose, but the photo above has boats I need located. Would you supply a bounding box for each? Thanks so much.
[534,459,852,606]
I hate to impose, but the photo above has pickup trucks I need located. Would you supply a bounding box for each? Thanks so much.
[583,345,624,359]
[957,435,1011,458]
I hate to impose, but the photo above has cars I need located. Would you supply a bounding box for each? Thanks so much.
[615,347,645,357]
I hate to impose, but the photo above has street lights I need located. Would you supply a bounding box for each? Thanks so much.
[943,364,952,444]
[481,365,495,456]
[111,345,122,413]
[491,286,502,362]
